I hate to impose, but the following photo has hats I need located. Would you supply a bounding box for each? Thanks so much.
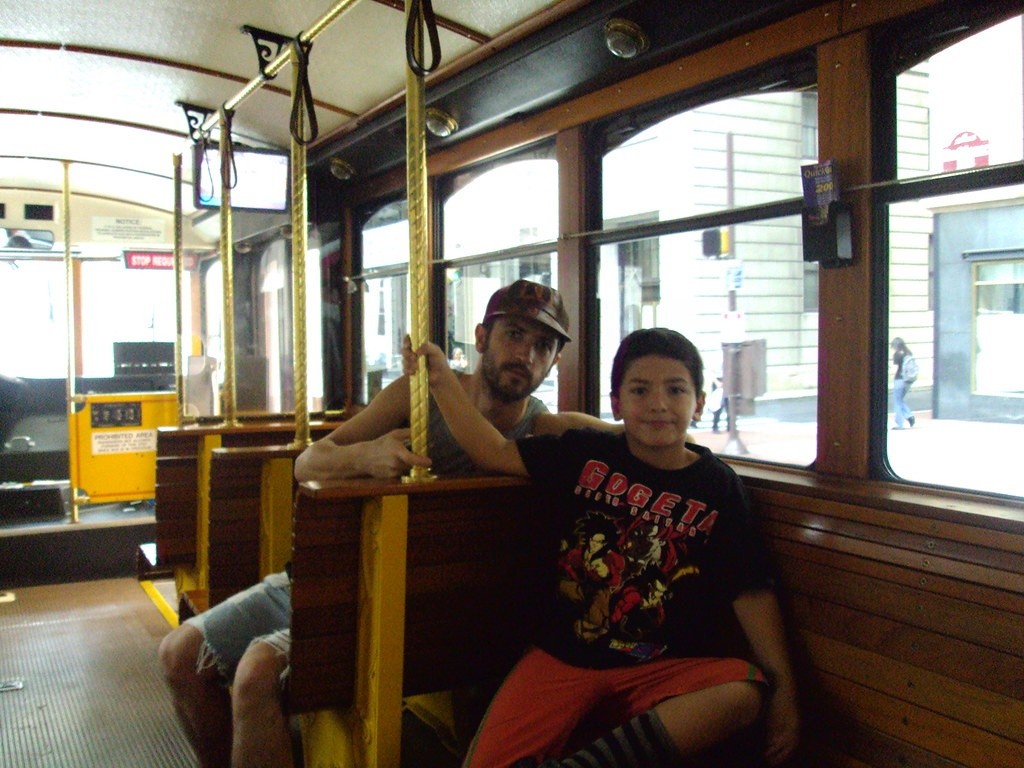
[482,279,573,344]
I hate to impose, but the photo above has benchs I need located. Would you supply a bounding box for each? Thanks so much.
[135,412,1023,768]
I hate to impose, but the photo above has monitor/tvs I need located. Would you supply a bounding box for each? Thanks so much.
[192,145,291,215]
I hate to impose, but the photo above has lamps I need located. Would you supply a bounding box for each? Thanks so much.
[424,107,458,138]
[232,240,252,254]
[603,17,651,60]
[327,158,359,181]
[278,223,292,239]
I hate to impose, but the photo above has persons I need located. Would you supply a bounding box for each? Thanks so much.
[889,337,915,430]
[447,347,468,373]
[401,327,798,768]
[159,280,572,768]
